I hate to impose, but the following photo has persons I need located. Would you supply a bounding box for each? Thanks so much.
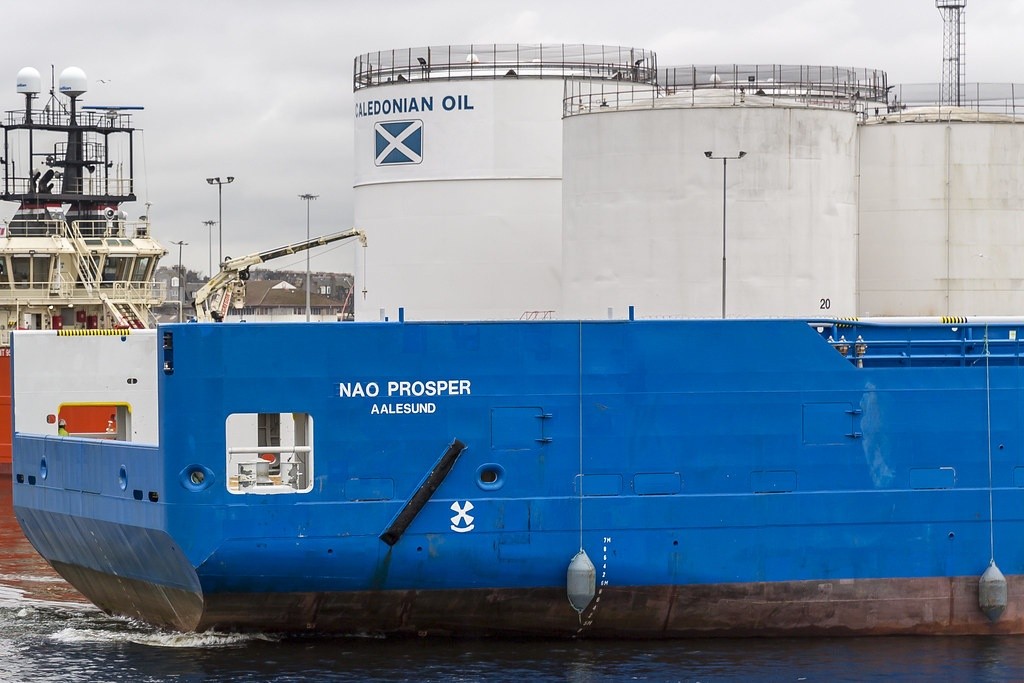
[58,419,69,437]
[137,216,147,238]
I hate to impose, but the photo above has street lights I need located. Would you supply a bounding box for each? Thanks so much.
[201,220,219,281]
[206,176,235,275]
[172,241,188,323]
[704,151,749,318]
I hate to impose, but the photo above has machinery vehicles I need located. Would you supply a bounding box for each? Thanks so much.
[191,226,370,327]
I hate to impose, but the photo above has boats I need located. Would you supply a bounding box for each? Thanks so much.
[0,66,176,479]
[10,41,1024,634]
